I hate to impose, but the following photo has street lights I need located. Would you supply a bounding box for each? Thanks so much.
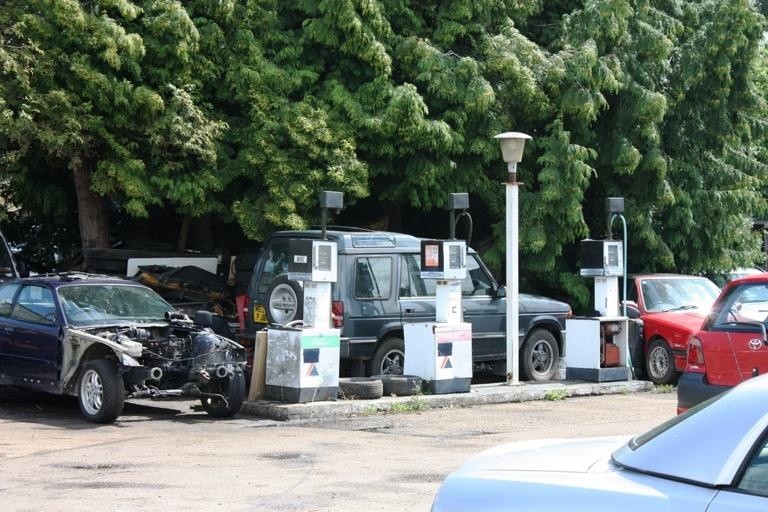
[495,131,534,385]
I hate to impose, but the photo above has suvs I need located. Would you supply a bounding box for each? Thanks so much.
[676,273,768,418]
[250,228,573,389]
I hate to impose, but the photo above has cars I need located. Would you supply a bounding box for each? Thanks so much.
[0,230,19,281]
[726,264,768,321]
[432,373,768,512]
[0,270,250,420]
[622,271,743,387]
